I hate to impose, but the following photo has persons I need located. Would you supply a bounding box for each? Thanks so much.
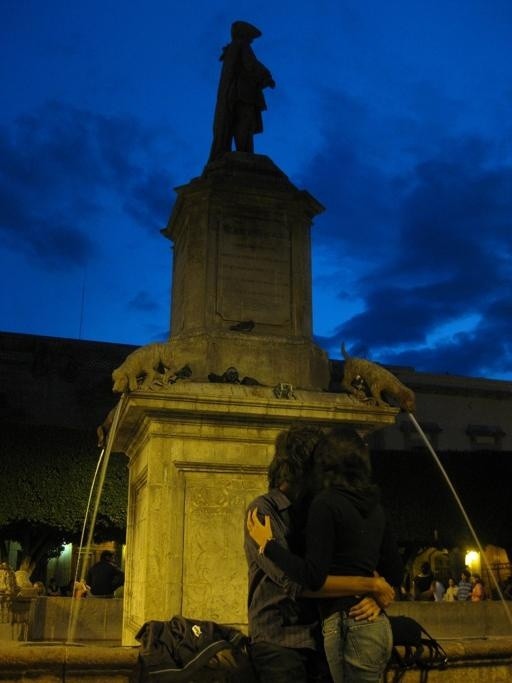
[420,572,445,602]
[209,19,276,155]
[63,577,74,596]
[46,577,59,595]
[443,576,458,601]
[467,574,484,602]
[85,549,124,595]
[458,569,472,600]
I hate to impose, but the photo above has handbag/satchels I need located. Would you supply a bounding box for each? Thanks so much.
[388,616,422,646]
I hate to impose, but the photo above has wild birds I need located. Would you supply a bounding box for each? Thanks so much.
[174,362,192,381]
[229,320,255,333]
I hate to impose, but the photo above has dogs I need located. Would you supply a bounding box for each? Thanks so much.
[340,341,416,413]
[112,343,178,391]
